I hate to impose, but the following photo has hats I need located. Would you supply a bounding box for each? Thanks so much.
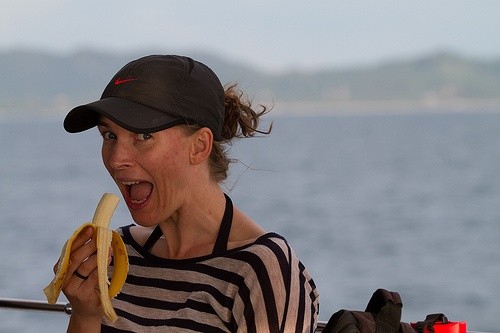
[61,55,226,137]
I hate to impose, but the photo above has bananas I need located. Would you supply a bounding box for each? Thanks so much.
[43,193,130,325]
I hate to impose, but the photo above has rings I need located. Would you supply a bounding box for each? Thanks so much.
[73,268,88,282]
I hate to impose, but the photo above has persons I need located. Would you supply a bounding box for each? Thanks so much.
[50,52,321,333]
